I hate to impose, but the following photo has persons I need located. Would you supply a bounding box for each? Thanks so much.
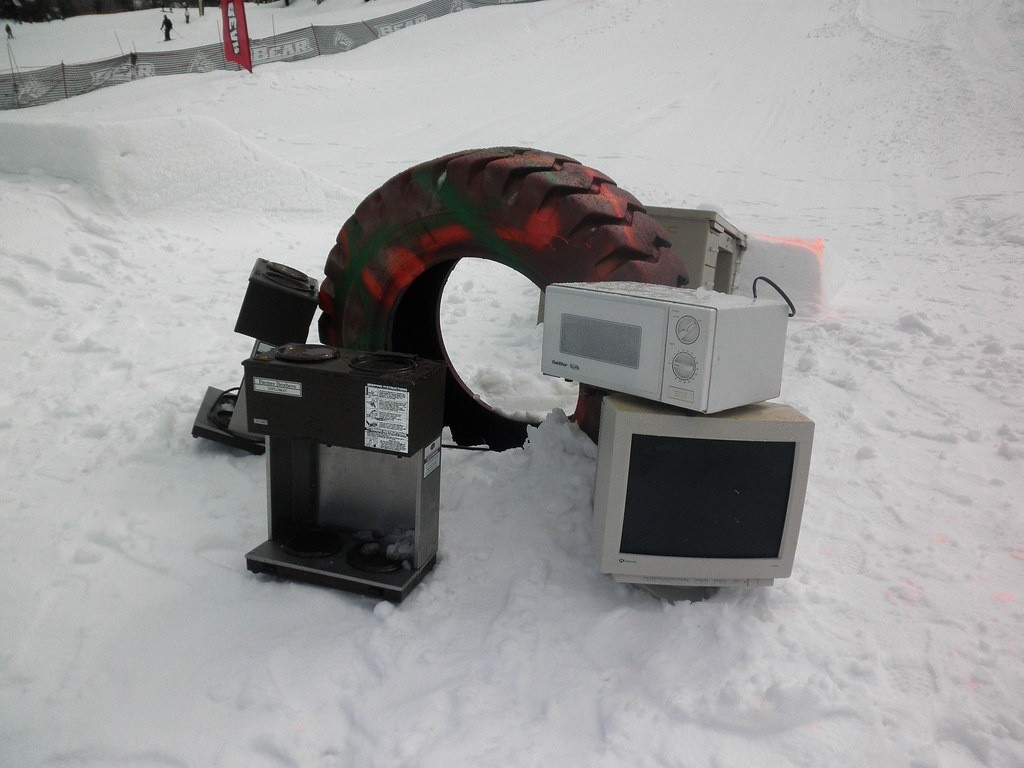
[159,14,173,42]
[5,23,14,40]
[185,9,189,23]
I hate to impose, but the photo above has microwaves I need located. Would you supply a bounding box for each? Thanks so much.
[540,281,788,414]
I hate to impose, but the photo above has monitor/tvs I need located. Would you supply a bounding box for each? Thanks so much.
[589,394,815,588]
